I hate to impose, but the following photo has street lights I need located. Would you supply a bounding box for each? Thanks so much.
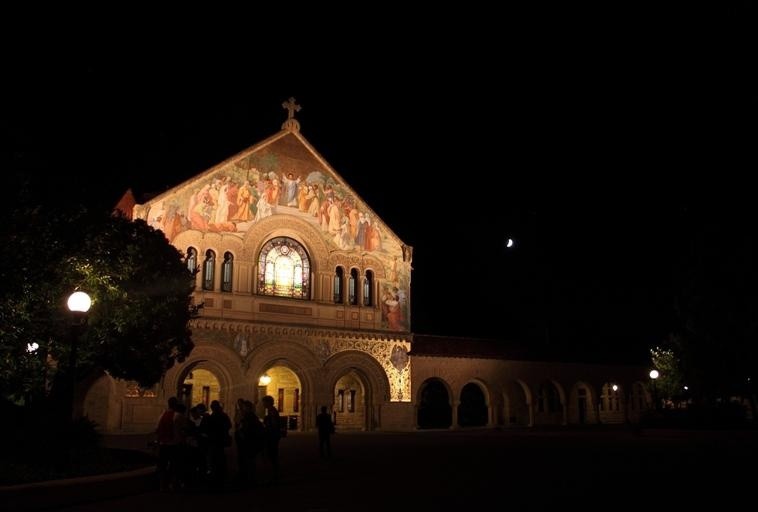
[65,283,93,477]
[650,369,659,408]
[26,333,40,445]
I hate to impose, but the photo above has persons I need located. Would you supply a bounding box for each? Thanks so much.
[381,286,398,317]
[316,406,336,464]
[158,166,394,250]
[152,394,281,488]
[384,294,403,330]
